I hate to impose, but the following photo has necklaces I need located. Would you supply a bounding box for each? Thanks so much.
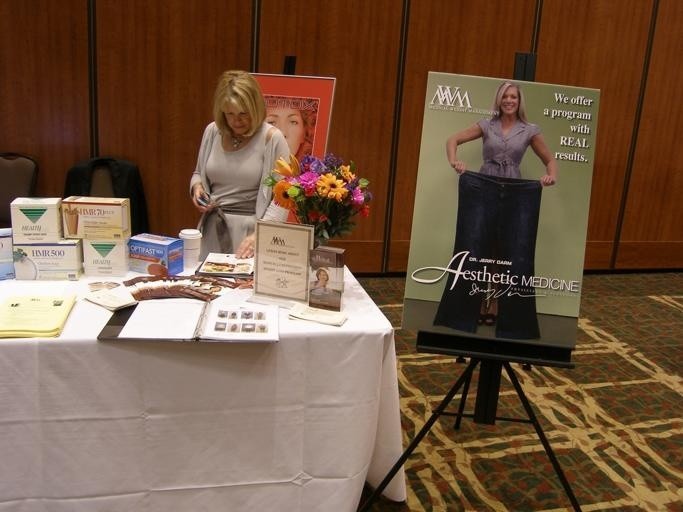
[232,136,241,148]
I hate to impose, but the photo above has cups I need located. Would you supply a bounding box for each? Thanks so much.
[64,211,77,236]
[178,228,202,269]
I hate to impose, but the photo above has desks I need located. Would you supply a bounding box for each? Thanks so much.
[0,247,407,512]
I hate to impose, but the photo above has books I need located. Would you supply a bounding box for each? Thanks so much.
[118,297,280,344]
[194,251,254,279]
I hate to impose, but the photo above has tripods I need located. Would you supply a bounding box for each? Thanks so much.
[358,359,584,512]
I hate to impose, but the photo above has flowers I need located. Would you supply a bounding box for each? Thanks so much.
[262,149,375,244]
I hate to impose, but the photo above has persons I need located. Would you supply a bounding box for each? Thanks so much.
[189,71,294,260]
[308,267,331,295]
[445,80,557,327]
[260,100,310,159]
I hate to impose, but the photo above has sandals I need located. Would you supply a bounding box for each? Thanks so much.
[486,313,498,328]
[477,313,486,326]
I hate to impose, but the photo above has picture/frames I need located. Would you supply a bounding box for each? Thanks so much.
[247,73,337,165]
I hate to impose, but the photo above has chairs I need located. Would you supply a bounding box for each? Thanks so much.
[65,156,149,239]
[0,152,38,226]
